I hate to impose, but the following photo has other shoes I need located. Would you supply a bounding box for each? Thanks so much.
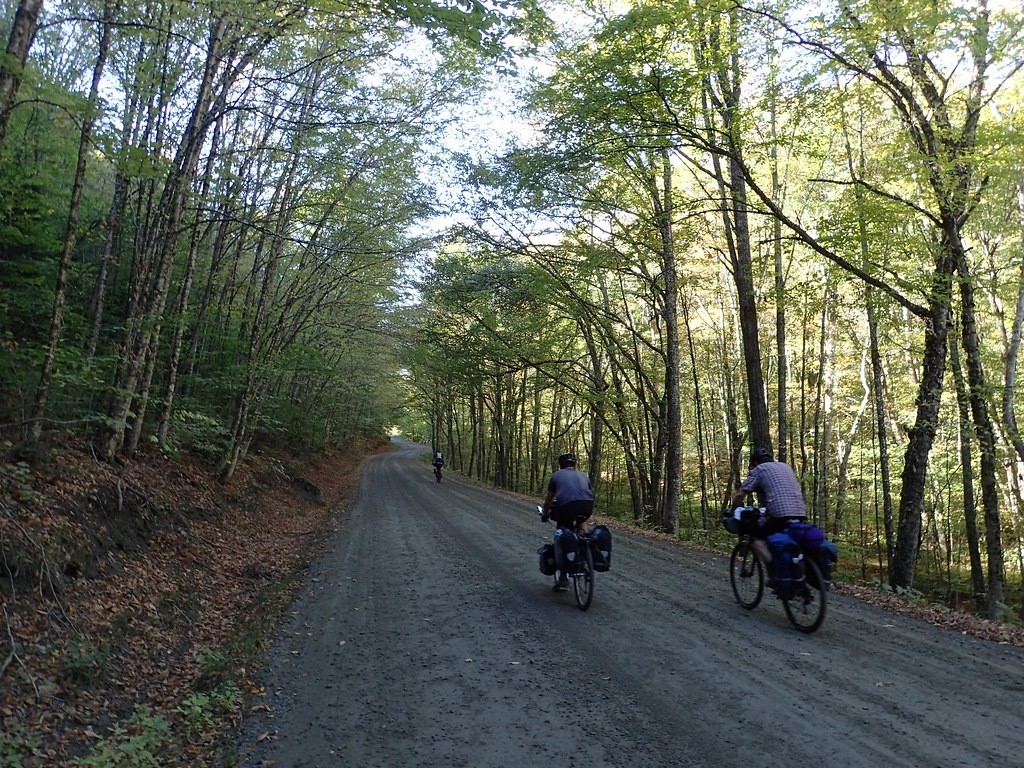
[552,580,568,589]
[772,580,794,594]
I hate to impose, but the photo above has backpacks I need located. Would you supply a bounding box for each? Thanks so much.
[436,452,442,459]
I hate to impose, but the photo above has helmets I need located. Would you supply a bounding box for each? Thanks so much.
[558,453,576,464]
[749,447,773,461]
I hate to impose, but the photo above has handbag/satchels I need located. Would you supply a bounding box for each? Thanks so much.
[591,524,613,572]
[537,543,557,575]
[553,526,587,573]
[783,521,836,591]
[765,532,799,590]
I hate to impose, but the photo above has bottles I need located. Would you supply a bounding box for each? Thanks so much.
[746,506,753,510]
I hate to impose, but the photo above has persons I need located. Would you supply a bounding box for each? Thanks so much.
[732,447,808,588]
[544,453,594,587]
[433,450,444,474]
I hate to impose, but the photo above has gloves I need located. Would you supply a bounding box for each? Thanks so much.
[541,513,549,522]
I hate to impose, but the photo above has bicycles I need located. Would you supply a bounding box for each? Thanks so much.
[536,499,595,611]
[432,463,444,483]
[722,507,830,634]
